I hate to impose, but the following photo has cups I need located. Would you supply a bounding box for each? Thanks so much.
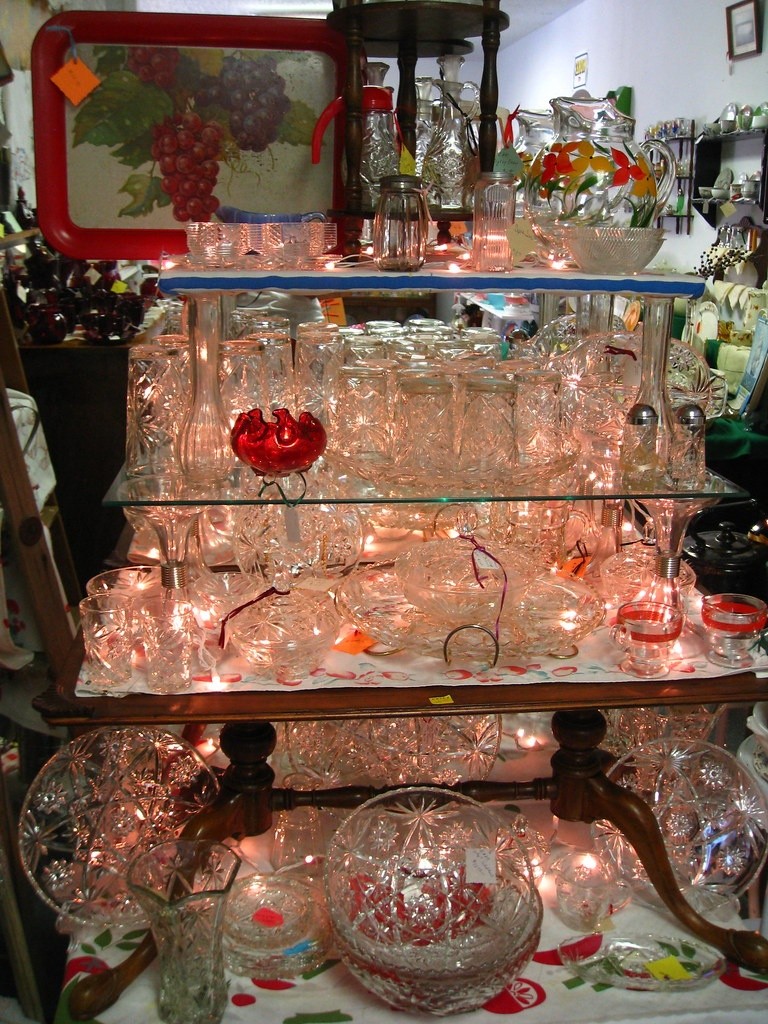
[702,116,768,137]
[610,593,768,678]
[190,572,265,651]
[79,477,194,694]
[126,308,708,496]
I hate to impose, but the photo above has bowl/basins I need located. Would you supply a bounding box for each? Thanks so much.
[226,538,537,679]
[557,224,668,276]
[698,181,759,198]
[182,221,337,270]
[326,786,543,1018]
[599,550,696,606]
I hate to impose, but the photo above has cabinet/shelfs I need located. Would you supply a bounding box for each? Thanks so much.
[691,128,768,225]
[30,273,765,722]
[649,119,695,236]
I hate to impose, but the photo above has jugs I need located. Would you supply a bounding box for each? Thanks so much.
[272,772,327,877]
[313,47,677,264]
[552,853,632,932]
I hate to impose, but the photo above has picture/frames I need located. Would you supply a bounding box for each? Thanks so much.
[726,0,761,60]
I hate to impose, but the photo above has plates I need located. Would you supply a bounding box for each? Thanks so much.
[556,933,728,991]
[18,711,766,967]
[717,102,768,125]
[335,556,607,660]
[713,168,761,189]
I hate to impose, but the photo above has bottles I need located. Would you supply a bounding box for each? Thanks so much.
[372,174,428,273]
[507,812,550,889]
[471,172,517,273]
[718,224,743,250]
[126,839,243,1023]
